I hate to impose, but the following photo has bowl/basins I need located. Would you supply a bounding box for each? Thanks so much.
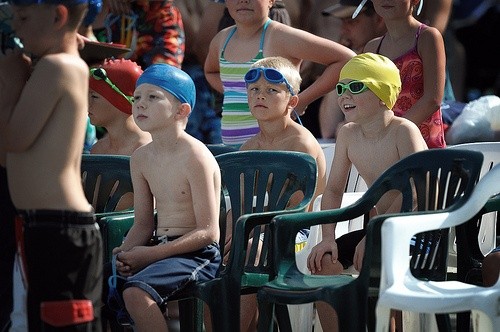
[80,41,131,68]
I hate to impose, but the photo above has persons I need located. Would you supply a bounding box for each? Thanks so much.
[105,63,222,331]
[322,1,389,58]
[71,1,322,143]
[205,1,359,151]
[83,58,156,213]
[199,57,327,330]
[308,53,440,332]
[364,0,454,150]
[0,0,100,330]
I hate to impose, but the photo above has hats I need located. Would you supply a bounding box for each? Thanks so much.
[135,62,196,116]
[89,59,144,115]
[339,52,402,109]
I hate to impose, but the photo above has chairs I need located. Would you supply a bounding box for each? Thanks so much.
[374,161,500,331]
[91,149,321,332]
[241,147,484,332]
[81,154,132,244]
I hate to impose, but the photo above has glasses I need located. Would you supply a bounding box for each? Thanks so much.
[335,80,369,96]
[89,66,134,104]
[244,67,294,97]
